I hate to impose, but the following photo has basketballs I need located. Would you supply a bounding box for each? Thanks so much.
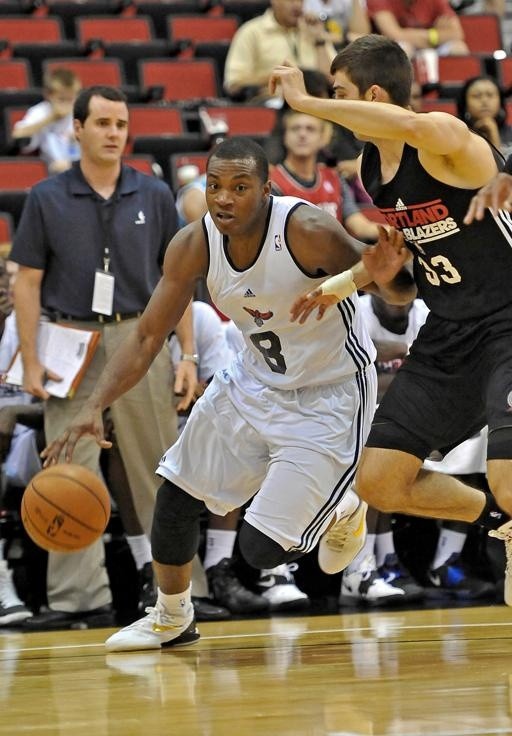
[21,463,111,554]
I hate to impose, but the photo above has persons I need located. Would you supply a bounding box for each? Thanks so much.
[0,1,512,652]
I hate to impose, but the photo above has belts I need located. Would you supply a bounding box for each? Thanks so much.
[60,311,143,325]
